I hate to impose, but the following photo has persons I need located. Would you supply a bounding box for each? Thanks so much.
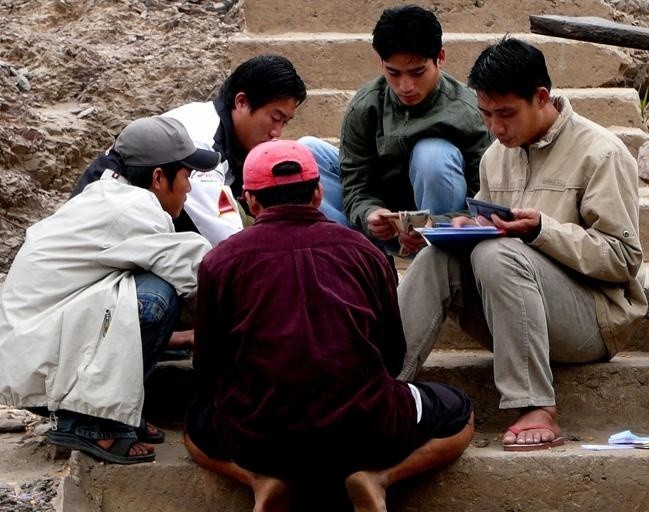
[183,135,477,511]
[62,54,307,250]
[391,30,647,453]
[295,3,493,258]
[1,112,223,464]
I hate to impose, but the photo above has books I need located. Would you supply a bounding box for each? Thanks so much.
[413,225,506,259]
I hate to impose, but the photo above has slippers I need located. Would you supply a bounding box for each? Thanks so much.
[48,418,165,463]
[504,426,565,451]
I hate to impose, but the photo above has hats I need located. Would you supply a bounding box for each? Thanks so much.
[115,115,221,172]
[243,139,319,192]
